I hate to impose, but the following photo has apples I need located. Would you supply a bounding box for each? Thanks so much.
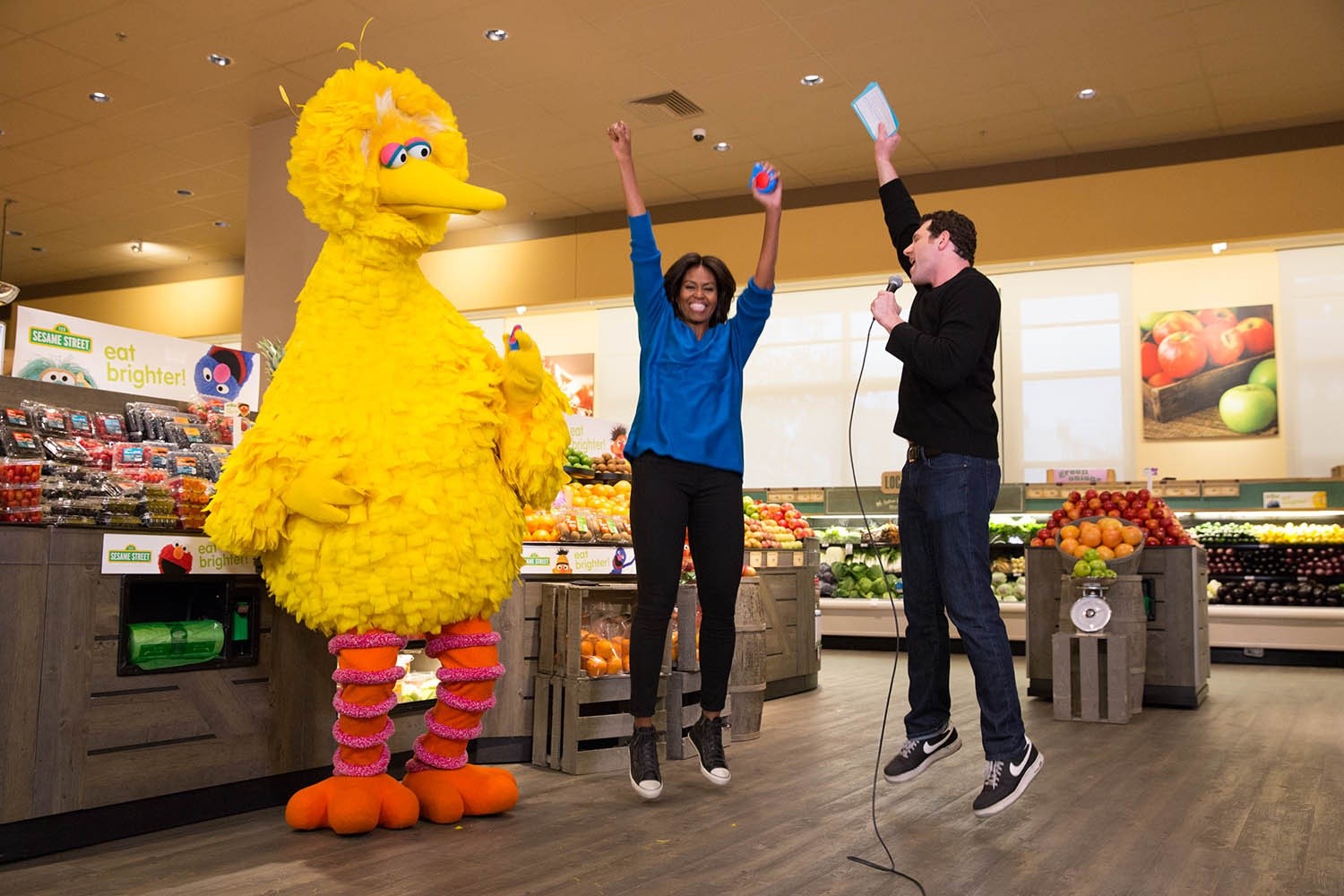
[1031,491,1203,546]
[1069,551,1116,577]
[1141,310,1277,431]
[743,495,815,548]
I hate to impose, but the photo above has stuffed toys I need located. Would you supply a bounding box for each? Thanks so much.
[15,354,99,390]
[549,546,637,576]
[610,423,630,460]
[194,345,256,405]
[159,544,194,576]
[196,16,572,838]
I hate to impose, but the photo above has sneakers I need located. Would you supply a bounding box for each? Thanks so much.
[883,722,963,783]
[688,710,732,786]
[627,722,664,799]
[973,736,1045,817]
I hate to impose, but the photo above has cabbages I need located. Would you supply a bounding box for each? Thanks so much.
[832,560,897,599]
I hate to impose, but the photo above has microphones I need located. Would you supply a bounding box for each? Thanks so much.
[872,274,904,321]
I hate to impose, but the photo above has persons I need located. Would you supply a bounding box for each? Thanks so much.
[871,120,1043,820]
[606,117,780,806]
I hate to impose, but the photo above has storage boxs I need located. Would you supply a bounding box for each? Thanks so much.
[666,672,732,762]
[674,576,701,673]
[531,673,671,772]
[543,580,677,675]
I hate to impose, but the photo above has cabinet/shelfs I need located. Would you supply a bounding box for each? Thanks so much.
[798,509,1344,659]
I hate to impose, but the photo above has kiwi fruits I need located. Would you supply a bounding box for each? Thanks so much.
[556,514,630,542]
[593,453,629,475]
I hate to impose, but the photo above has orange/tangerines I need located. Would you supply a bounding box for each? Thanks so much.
[564,445,591,470]
[514,480,631,540]
[579,622,700,680]
[1060,518,1141,561]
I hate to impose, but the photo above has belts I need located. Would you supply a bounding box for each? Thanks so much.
[907,445,943,463]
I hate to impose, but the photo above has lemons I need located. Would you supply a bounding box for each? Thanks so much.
[1256,517,1343,541]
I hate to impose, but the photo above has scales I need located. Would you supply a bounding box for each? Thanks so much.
[1068,576,1118,637]
